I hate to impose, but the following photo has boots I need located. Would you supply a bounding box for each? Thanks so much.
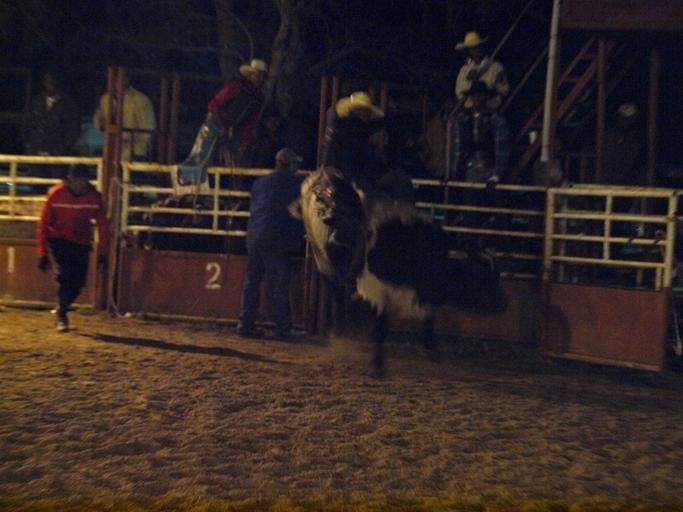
[57,308,69,332]
[54,305,60,318]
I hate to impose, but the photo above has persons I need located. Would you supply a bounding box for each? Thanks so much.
[450,81,513,271]
[20,71,80,194]
[347,130,448,374]
[236,148,306,340]
[95,65,155,222]
[322,91,384,171]
[455,31,509,112]
[420,96,454,179]
[172,59,268,195]
[39,165,108,331]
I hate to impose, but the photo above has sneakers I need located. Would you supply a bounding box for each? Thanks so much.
[236,318,253,336]
[278,324,297,340]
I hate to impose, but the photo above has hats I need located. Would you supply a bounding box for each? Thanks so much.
[334,92,382,119]
[239,58,270,76]
[460,79,497,100]
[453,30,489,50]
[276,147,303,164]
[65,163,93,180]
